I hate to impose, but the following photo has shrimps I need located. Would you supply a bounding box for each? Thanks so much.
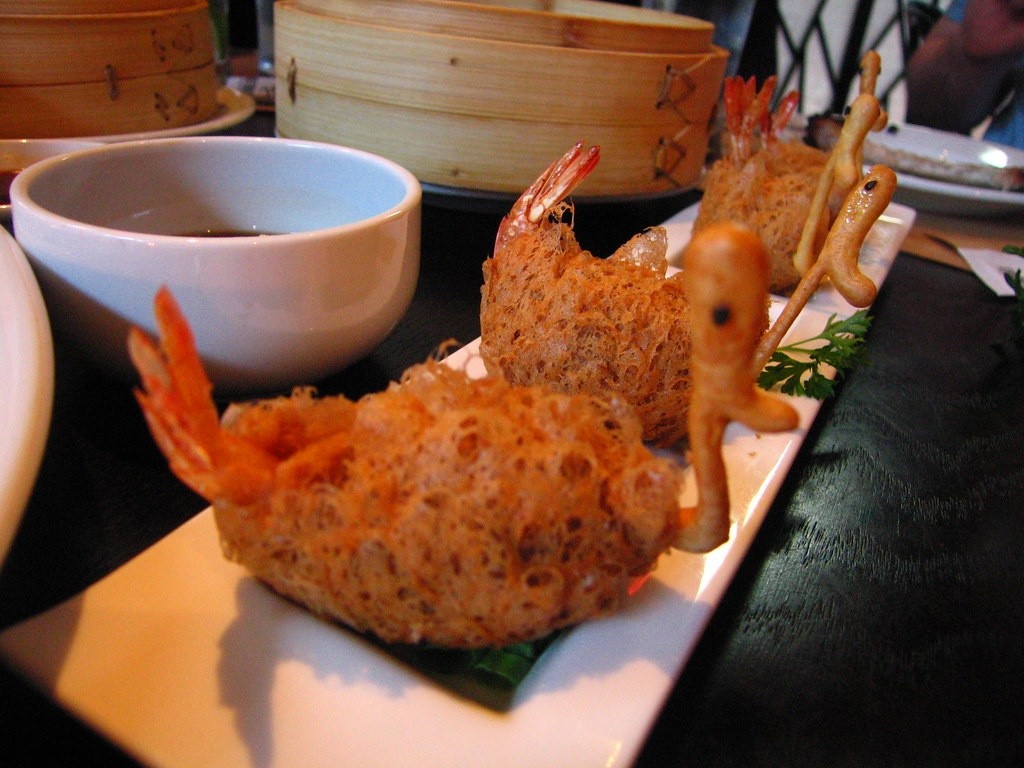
[131,61,800,505]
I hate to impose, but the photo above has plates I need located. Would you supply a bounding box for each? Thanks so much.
[813,115,1024,192]
[0,226,54,576]
[831,156,1024,216]
[0,87,258,141]
[0,198,916,768]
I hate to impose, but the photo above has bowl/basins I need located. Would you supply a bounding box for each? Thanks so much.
[11,138,422,389]
[0,136,104,222]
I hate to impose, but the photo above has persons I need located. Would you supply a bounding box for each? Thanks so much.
[905,0,1024,148]
